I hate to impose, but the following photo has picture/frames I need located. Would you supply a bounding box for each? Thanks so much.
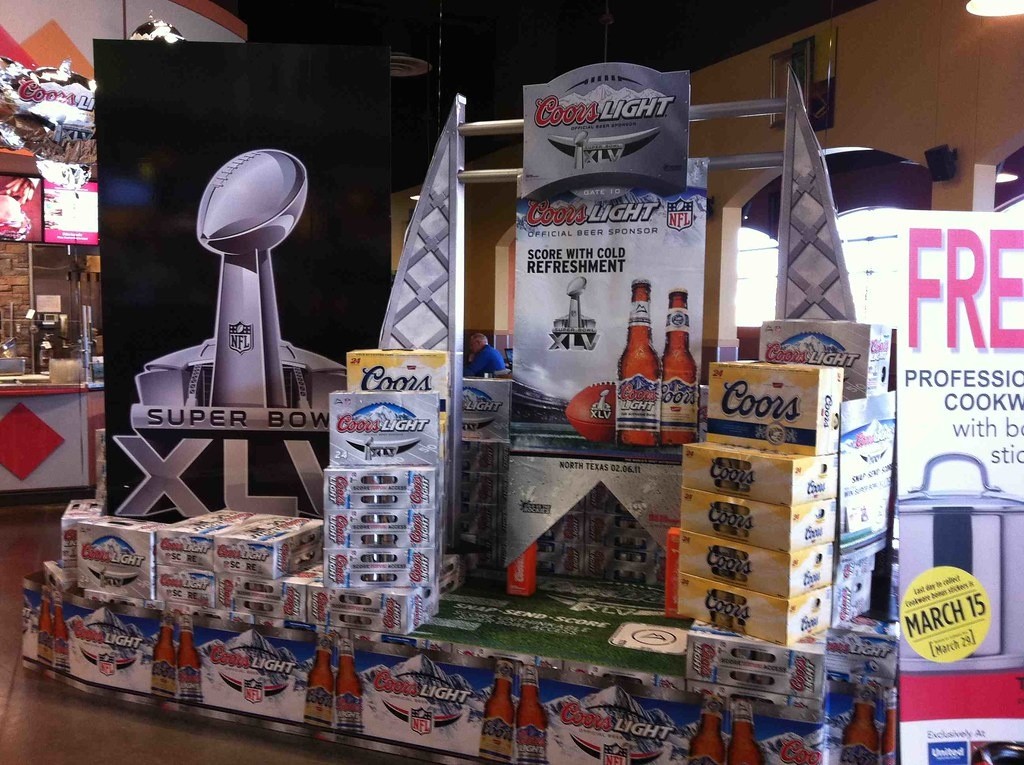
[0,171,44,244]
[769,41,809,128]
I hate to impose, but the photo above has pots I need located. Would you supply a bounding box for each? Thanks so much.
[900,450,1024,678]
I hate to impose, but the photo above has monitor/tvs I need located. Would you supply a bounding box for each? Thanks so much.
[0,172,98,247]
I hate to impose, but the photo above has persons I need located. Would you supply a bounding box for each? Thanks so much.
[462,333,508,378]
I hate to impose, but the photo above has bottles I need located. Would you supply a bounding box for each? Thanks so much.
[52,593,70,675]
[178,612,203,704]
[514,663,550,765]
[688,690,727,764]
[613,276,662,450]
[657,283,699,449]
[477,656,515,765]
[302,633,334,727]
[35,586,53,670]
[840,679,880,765]
[882,685,902,765]
[336,638,363,734]
[148,613,176,701]
[727,694,767,765]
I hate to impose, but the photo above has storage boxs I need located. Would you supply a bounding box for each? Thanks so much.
[47,320,902,708]
[0,357,27,373]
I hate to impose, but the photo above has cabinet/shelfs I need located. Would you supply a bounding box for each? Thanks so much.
[0,381,93,507]
[87,379,105,488]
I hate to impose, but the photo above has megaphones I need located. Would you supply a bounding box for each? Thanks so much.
[924,145,955,181]
